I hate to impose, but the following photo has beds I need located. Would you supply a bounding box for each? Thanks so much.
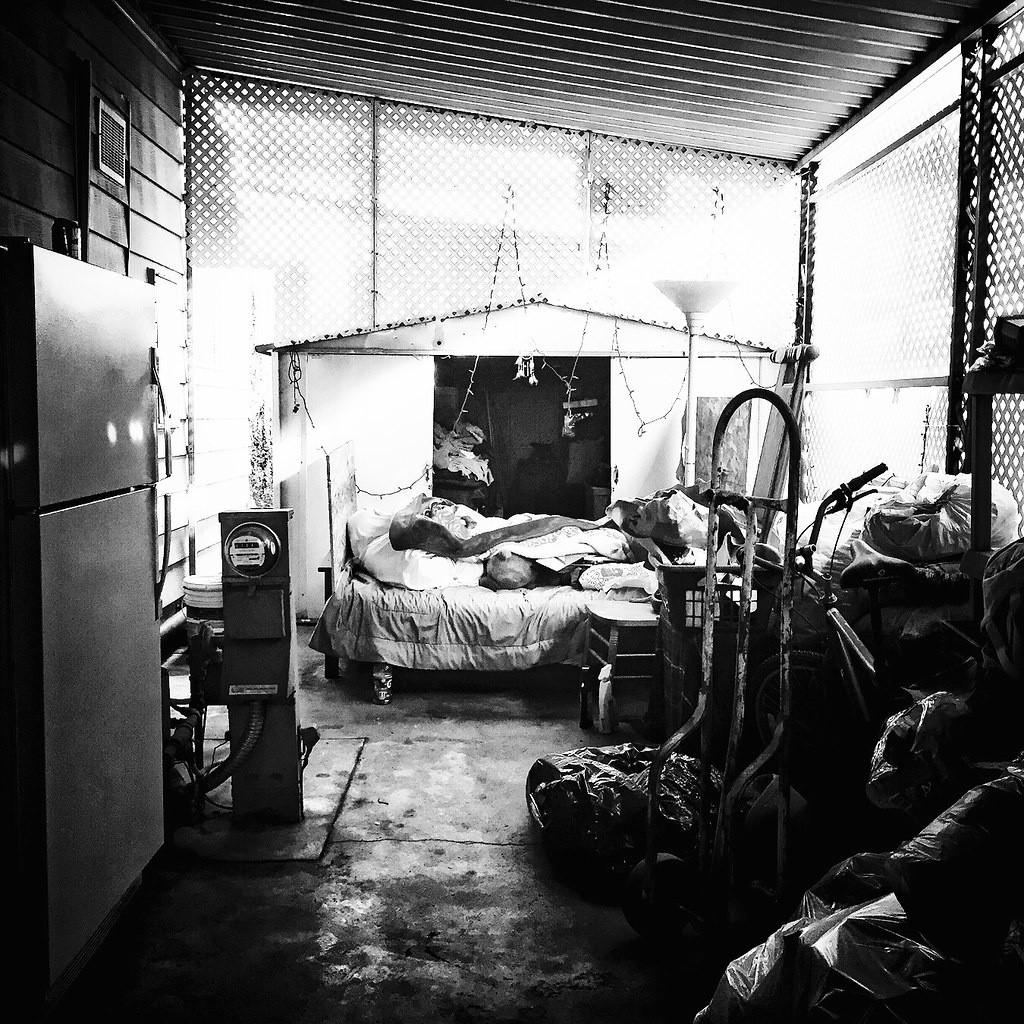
[309,546,756,684]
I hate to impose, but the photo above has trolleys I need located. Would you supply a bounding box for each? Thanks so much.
[620,388,804,953]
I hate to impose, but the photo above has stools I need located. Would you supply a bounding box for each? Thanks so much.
[580,601,660,732]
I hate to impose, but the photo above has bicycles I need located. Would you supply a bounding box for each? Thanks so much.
[736,463,951,850]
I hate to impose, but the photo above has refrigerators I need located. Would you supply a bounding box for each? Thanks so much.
[1,242,172,987]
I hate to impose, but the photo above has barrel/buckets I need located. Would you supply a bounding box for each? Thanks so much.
[181,572,224,701]
